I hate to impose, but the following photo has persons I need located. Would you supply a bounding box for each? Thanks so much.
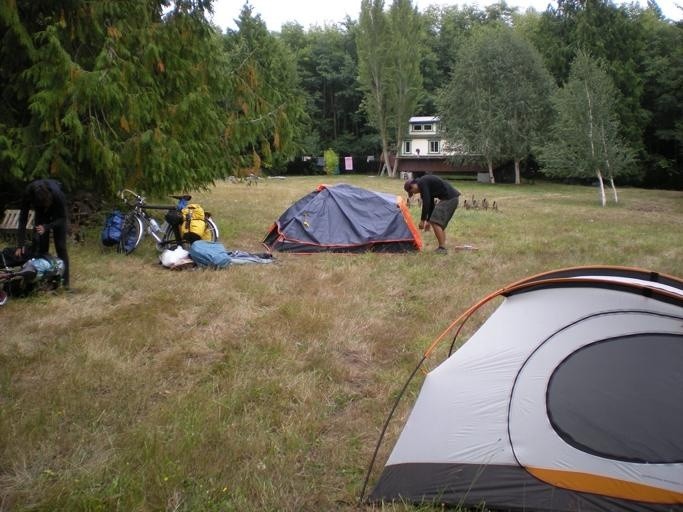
[14,177,70,292]
[404,174,461,255]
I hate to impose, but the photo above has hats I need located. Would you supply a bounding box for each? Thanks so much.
[404,180,413,197]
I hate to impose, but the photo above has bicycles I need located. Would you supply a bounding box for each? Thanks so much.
[0,251,64,307]
[109,186,220,260]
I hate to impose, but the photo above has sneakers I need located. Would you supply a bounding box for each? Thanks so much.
[434,247,445,254]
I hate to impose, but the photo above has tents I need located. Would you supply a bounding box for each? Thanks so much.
[355,266,682,512]
[259,183,424,255]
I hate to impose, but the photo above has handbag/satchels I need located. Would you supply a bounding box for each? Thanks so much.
[181,204,211,242]
[101,212,142,249]
[4,232,70,289]
